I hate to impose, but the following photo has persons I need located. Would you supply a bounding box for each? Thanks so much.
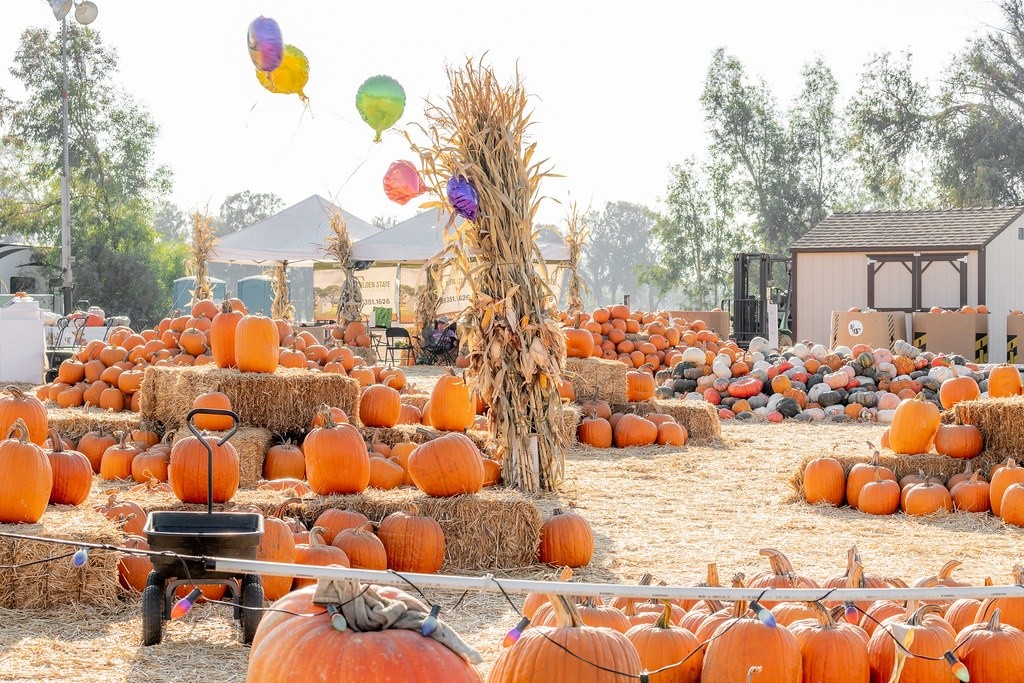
[420,317,455,352]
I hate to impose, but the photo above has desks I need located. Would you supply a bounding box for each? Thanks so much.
[370,335,382,362]
[366,328,387,364]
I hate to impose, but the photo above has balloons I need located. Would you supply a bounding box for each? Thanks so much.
[355,74,407,143]
[254,44,310,103]
[382,160,431,205]
[446,176,481,223]
[247,16,283,80]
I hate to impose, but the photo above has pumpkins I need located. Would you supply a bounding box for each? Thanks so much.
[0,298,1024,683]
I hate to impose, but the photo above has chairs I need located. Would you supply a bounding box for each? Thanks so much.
[412,321,462,367]
[385,328,415,365]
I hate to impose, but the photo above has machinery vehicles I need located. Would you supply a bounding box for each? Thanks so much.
[719,250,793,354]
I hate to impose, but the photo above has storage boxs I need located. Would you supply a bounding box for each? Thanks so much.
[0,292,43,384]
[911,310,989,366]
[1006,310,1024,364]
[831,310,907,356]
[670,311,730,345]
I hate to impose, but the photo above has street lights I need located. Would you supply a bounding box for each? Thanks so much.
[47,0,100,318]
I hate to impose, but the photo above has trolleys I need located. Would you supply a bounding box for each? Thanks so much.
[140,406,264,648]
